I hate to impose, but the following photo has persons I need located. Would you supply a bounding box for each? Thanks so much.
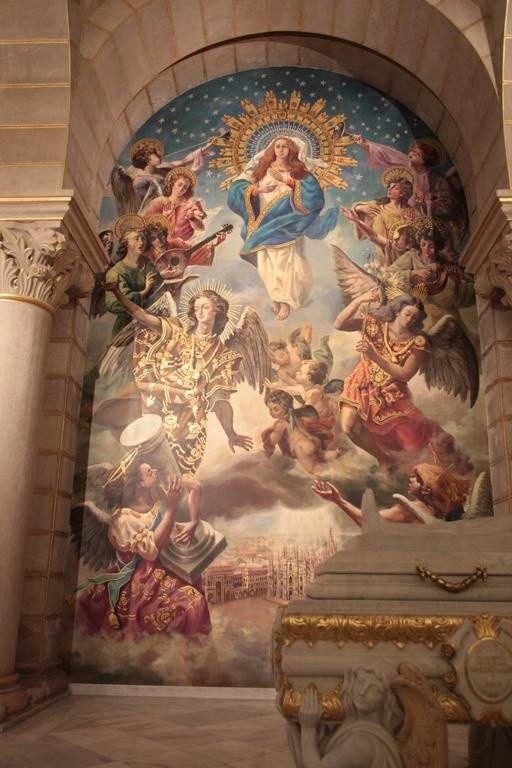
[274,651,449,766]
